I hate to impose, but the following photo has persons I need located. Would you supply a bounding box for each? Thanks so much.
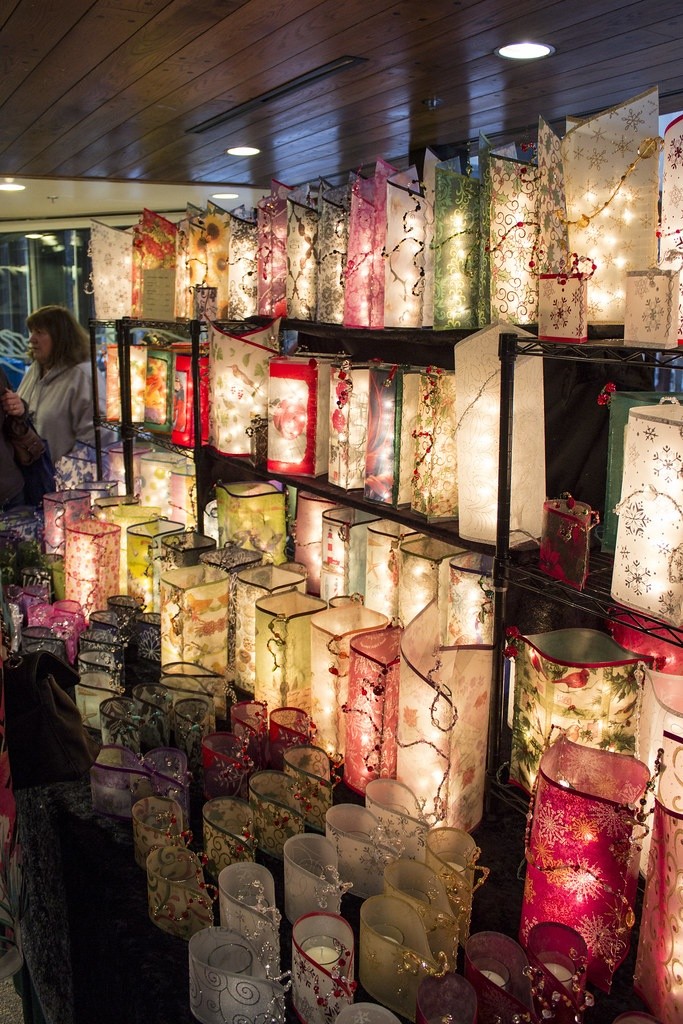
[0,366,47,514]
[15,306,118,469]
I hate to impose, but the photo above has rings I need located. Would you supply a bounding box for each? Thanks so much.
[13,404,17,410]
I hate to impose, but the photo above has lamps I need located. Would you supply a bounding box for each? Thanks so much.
[1,81,683,1024]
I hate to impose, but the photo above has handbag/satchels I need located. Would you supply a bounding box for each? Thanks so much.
[5,385,60,507]
